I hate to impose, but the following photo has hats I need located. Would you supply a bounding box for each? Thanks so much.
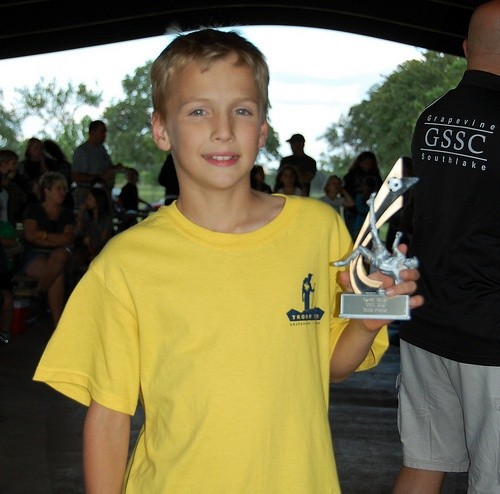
[286,134,306,144]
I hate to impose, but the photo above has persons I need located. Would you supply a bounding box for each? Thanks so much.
[385,0,500,494]
[250,134,413,275]
[0,120,151,328]
[156,153,181,204]
[31,28,424,494]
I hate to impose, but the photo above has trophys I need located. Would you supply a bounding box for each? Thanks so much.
[333,192,418,320]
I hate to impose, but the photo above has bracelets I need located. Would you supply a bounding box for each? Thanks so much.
[43,231,47,239]
